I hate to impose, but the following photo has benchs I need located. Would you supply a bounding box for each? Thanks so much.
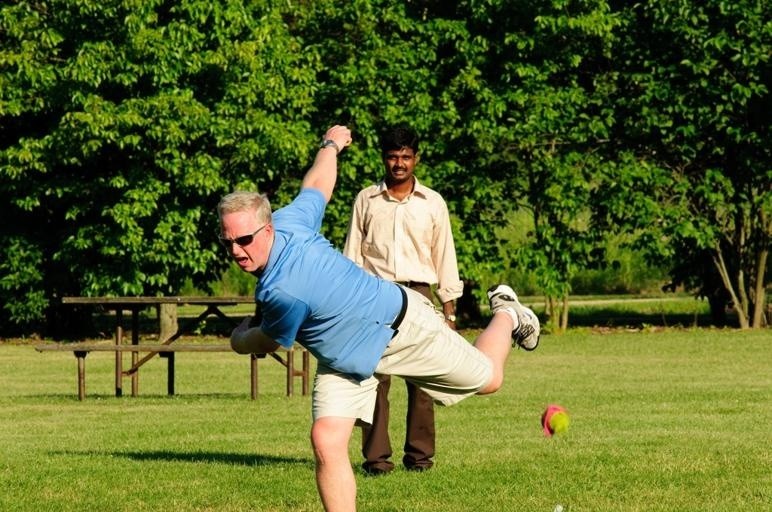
[34,340,310,402]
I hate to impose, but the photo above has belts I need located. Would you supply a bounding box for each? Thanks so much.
[387,277,432,346]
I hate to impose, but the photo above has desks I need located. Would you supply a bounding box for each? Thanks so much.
[59,296,310,396]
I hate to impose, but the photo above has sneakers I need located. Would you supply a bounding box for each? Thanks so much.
[486,282,541,352]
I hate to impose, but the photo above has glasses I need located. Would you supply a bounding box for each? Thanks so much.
[215,223,267,249]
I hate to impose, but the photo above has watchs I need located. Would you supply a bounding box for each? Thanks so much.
[444,312,457,322]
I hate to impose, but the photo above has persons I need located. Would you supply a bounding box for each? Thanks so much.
[216,124,542,510]
[344,123,466,475]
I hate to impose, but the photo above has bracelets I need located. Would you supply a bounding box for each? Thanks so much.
[319,139,339,154]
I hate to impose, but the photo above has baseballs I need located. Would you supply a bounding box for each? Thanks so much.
[540,407,570,432]
